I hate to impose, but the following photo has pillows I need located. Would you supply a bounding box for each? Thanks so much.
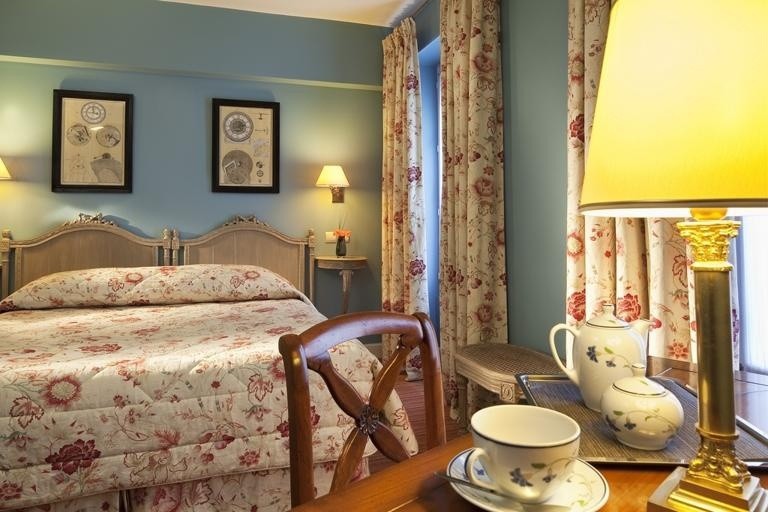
[1,262,300,311]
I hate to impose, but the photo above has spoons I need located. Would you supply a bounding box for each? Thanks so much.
[432,471,552,505]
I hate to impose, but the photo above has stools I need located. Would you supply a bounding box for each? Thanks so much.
[453,340,566,436]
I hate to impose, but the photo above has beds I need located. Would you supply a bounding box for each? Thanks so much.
[0,211,372,512]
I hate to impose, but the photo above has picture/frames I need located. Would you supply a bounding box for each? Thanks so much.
[211,97,281,194]
[51,88,135,194]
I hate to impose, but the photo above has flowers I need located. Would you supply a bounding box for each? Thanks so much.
[332,215,352,244]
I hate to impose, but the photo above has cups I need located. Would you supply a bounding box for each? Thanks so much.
[463,404,582,504]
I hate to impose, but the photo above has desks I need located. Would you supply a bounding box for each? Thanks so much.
[315,255,369,314]
[286,431,768,512]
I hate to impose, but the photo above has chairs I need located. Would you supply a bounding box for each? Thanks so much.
[276,311,448,508]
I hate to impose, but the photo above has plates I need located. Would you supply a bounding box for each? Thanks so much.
[446,446,610,512]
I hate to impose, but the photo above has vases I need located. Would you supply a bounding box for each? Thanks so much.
[336,236,347,256]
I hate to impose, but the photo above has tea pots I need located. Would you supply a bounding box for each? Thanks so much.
[548,302,657,414]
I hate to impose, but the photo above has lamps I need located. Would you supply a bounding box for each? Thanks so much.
[314,164,350,203]
[0,154,12,183]
[576,0,768,511]
